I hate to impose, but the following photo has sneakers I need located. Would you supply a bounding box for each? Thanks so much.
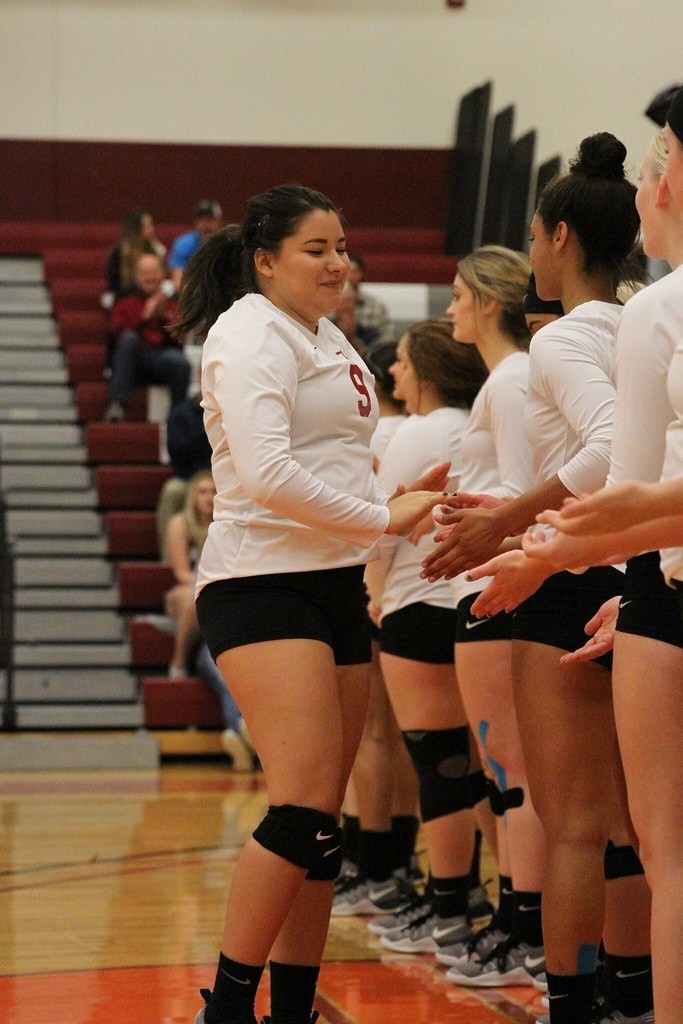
[434,905,510,966]
[380,910,472,953]
[536,1007,654,1023]
[541,993,549,1007]
[367,892,435,934]
[329,870,403,916]
[533,971,548,992]
[446,933,546,987]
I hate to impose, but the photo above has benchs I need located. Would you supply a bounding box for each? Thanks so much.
[2,219,461,732]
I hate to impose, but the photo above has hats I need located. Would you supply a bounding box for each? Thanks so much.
[192,196,223,220]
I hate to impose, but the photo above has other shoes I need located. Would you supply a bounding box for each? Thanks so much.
[102,369,113,380]
[169,667,187,682]
[105,409,124,423]
[194,1002,275,1024]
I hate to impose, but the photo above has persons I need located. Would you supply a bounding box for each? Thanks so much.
[164,469,215,679]
[335,82,683,1024]
[437,243,546,985]
[165,184,458,1024]
[98,211,167,376]
[168,197,226,293]
[324,255,390,355]
[106,252,189,424]
[194,644,259,772]
[363,318,481,954]
